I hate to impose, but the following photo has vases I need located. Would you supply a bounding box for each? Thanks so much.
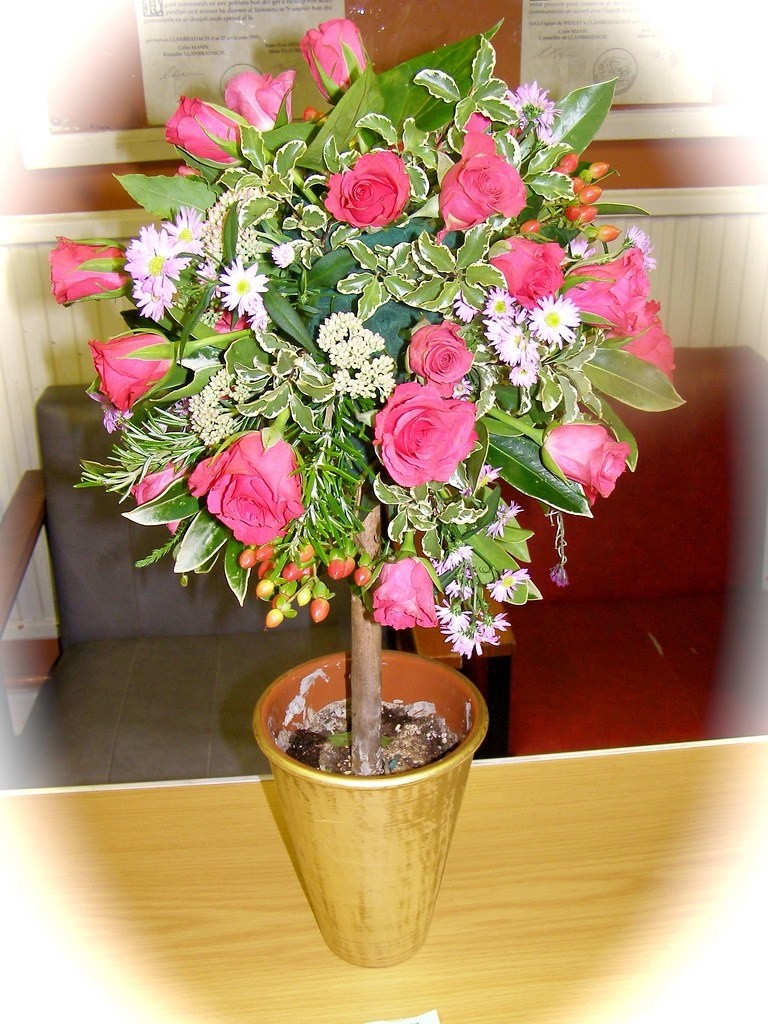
[250,648,490,969]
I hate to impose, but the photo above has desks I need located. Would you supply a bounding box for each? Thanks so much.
[0,735,764,1011]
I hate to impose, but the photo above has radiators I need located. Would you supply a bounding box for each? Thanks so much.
[0,181,768,639]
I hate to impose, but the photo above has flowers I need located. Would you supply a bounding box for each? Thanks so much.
[46,16,686,774]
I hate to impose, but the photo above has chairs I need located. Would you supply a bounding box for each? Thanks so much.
[470,339,768,755]
[0,384,464,790]
